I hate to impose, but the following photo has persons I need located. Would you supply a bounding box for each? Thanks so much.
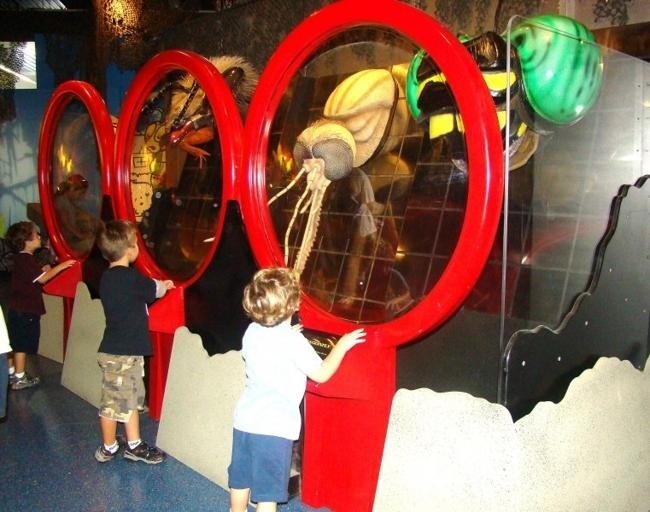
[3,221,77,390]
[0,304,14,422]
[336,238,413,314]
[54,175,96,243]
[93,219,178,466]
[226,266,368,512]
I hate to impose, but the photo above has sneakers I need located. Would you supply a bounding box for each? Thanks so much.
[12,375,39,389]
[7,373,19,385]
[124,443,165,465]
[94,437,128,463]
